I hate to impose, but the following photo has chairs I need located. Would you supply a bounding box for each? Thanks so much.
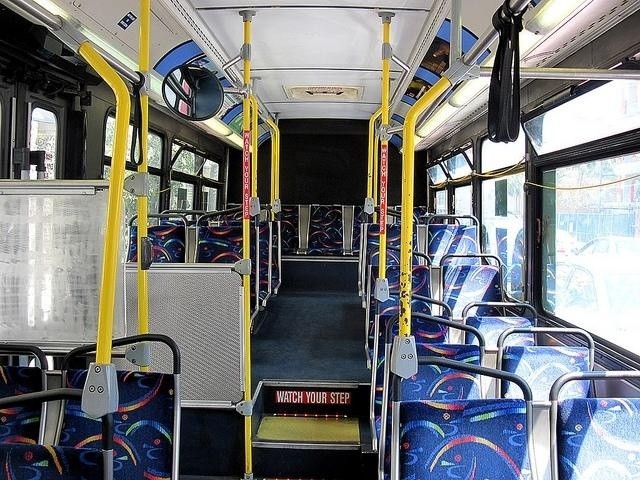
[125,206,282,335]
[0,334,185,480]
[265,202,431,261]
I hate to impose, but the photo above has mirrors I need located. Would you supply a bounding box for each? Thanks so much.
[160,64,224,119]
